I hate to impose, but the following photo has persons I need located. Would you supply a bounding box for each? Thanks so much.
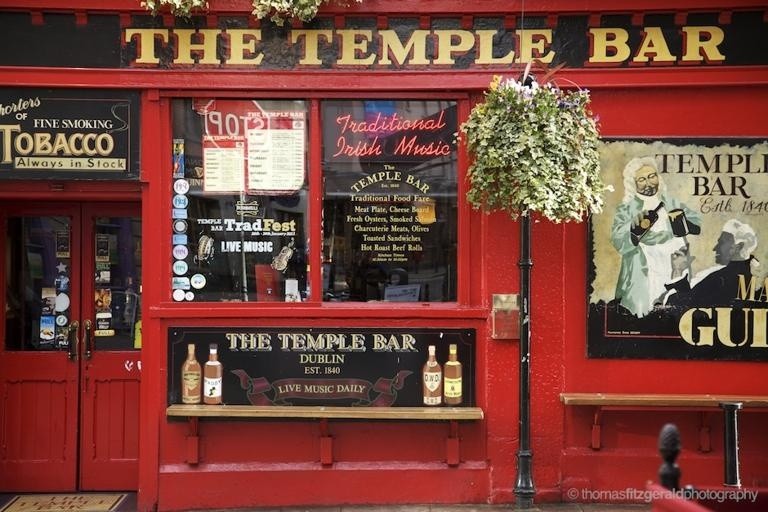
[645,216,760,315]
[604,154,702,317]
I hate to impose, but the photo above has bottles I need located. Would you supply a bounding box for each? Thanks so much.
[201,341,227,406]
[183,344,204,407]
[631,202,665,246]
[421,345,444,408]
[441,342,466,408]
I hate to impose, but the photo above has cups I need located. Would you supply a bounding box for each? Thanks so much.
[667,208,689,236]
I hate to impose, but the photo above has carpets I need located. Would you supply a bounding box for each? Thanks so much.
[0,494,128,512]
